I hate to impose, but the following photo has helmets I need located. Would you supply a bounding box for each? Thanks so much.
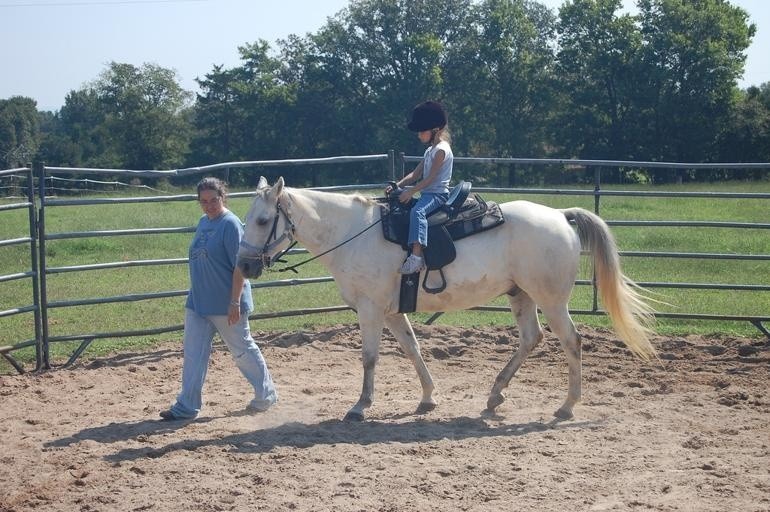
[407,99,447,133]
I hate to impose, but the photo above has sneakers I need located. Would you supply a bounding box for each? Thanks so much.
[395,255,426,276]
[247,405,267,415]
[159,410,176,420]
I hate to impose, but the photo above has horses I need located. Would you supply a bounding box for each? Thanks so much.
[235,174,681,422]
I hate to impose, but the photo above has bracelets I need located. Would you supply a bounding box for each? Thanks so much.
[230,300,240,307]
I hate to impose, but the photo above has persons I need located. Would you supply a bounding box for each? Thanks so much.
[158,176,280,422]
[382,99,454,278]
